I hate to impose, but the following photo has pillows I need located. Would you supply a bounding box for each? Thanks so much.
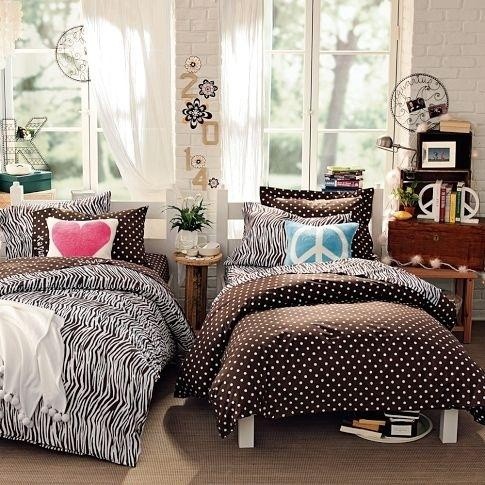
[31,204,149,269]
[223,201,353,267]
[0,190,112,261]
[259,186,377,260]
[273,196,363,217]
[280,219,359,266]
[45,216,120,260]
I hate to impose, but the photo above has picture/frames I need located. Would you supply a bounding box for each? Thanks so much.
[421,140,457,168]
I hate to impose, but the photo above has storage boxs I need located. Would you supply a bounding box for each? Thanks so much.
[388,217,485,272]
[0,171,52,193]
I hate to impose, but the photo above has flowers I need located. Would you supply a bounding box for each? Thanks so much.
[159,191,217,234]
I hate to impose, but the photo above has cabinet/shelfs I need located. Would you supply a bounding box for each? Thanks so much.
[399,130,473,215]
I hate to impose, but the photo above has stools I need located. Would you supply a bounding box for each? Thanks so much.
[401,266,478,344]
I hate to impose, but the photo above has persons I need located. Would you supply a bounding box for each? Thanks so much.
[433,152,439,159]
[440,151,446,160]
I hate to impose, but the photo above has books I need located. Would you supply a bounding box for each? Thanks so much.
[322,166,365,189]
[335,407,422,443]
[431,179,465,224]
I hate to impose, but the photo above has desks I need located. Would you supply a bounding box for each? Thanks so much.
[172,241,223,337]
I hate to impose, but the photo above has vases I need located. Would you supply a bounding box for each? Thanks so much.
[175,230,198,254]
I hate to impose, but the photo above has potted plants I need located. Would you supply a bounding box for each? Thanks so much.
[390,181,419,216]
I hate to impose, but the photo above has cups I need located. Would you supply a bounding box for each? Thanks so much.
[190,249,198,260]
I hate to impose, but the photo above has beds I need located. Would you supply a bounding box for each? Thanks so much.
[0,181,192,467]
[176,189,485,449]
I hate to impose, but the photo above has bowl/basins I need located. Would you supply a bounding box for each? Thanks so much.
[199,247,220,257]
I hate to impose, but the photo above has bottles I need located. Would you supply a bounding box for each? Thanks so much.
[9,180,23,207]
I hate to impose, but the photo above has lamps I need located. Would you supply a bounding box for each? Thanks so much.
[376,136,417,180]
